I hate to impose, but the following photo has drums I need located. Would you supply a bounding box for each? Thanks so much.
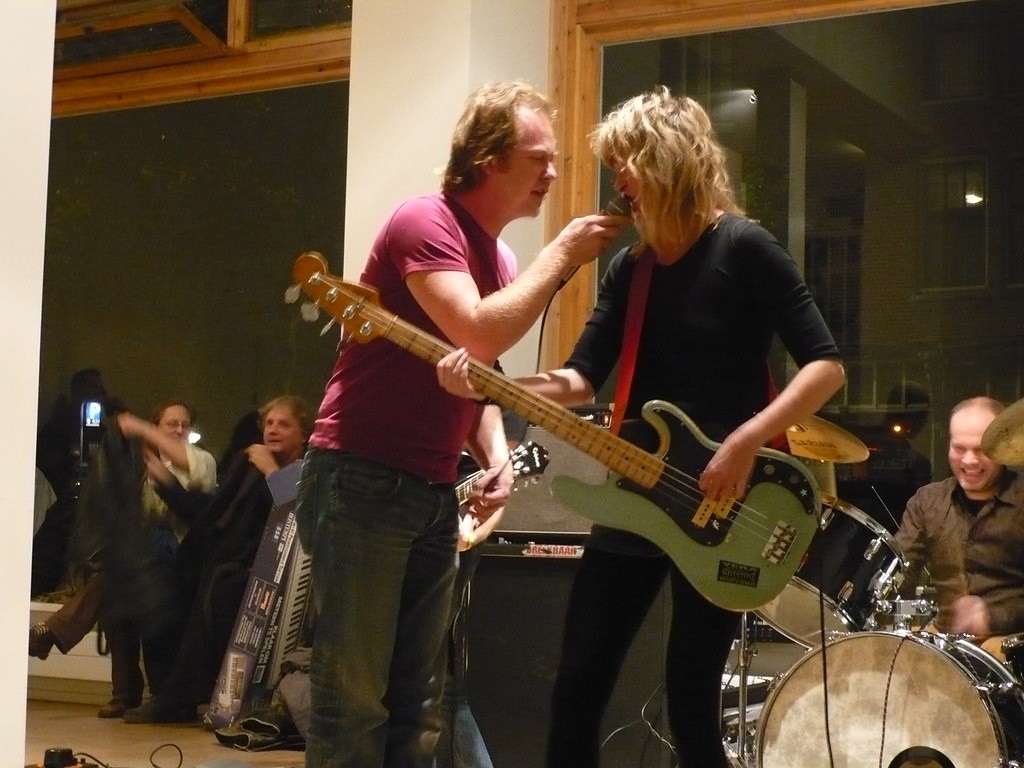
[751,492,910,650]
[754,628,1024,768]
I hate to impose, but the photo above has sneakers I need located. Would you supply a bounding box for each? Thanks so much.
[29,624,54,660]
[124,693,165,723]
[98,696,139,718]
[146,700,199,722]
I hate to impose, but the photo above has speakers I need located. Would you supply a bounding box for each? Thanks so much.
[442,529,677,768]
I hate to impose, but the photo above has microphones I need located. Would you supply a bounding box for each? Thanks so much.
[556,197,632,290]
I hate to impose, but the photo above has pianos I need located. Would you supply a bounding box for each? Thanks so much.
[266,532,314,691]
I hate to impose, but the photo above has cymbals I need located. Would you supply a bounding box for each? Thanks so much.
[980,396,1024,467]
[784,412,869,464]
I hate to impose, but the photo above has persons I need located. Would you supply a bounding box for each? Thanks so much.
[28,396,319,724]
[295,85,633,768]
[896,396,1023,678]
[435,82,846,768]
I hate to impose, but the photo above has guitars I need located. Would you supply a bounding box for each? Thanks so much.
[292,252,823,613]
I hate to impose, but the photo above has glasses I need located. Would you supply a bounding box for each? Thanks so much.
[608,161,637,184]
